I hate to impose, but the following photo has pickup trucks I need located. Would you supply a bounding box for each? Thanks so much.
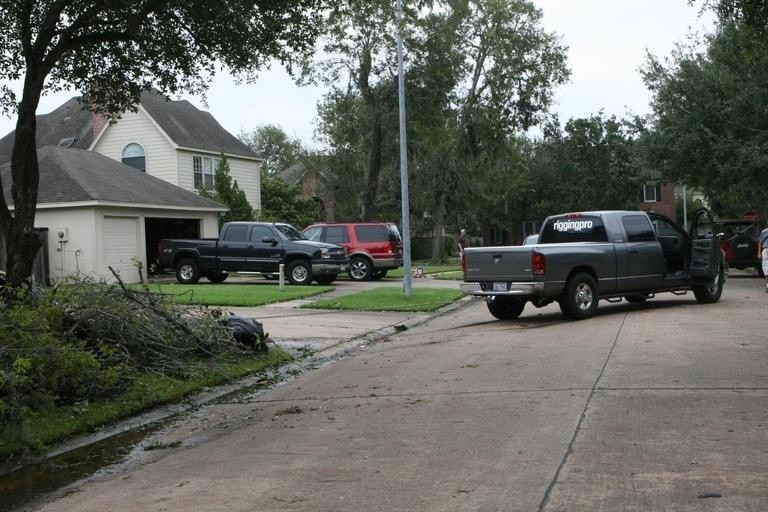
[157,220,351,286]
[459,206,724,320]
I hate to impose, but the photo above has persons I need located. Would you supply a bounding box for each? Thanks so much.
[757,228,768,293]
[456,228,471,259]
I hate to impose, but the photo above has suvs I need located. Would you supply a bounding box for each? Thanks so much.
[299,221,405,282]
[715,219,764,280]
